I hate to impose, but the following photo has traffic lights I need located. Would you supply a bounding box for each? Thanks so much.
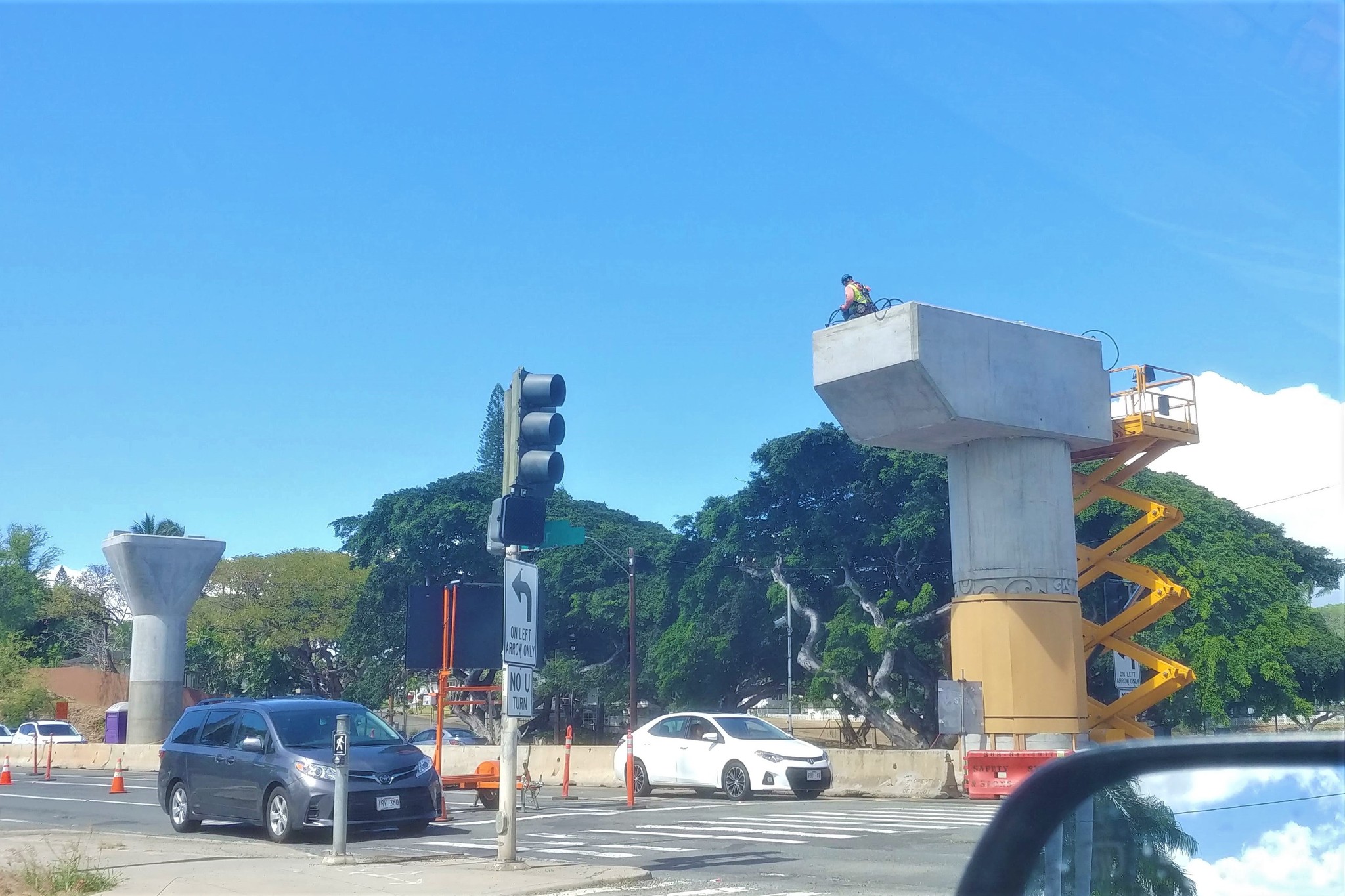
[518,368,564,498]
[489,494,546,550]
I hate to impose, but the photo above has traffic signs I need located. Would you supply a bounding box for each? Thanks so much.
[503,557,539,667]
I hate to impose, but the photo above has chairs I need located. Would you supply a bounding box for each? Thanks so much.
[690,724,707,741]
[659,725,670,735]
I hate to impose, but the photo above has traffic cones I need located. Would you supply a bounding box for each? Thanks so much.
[0,755,14,785]
[110,758,129,793]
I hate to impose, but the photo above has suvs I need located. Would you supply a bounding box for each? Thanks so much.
[157,697,441,844]
[12,720,88,744]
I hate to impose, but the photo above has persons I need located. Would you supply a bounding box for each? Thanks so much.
[689,723,705,741]
[839,274,876,322]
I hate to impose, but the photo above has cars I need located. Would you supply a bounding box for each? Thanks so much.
[614,712,834,801]
[408,727,489,745]
[0,724,13,744]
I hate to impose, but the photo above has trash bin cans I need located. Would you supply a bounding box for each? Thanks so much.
[105,702,128,744]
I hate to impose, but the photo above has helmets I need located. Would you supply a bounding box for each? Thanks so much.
[841,274,853,283]
